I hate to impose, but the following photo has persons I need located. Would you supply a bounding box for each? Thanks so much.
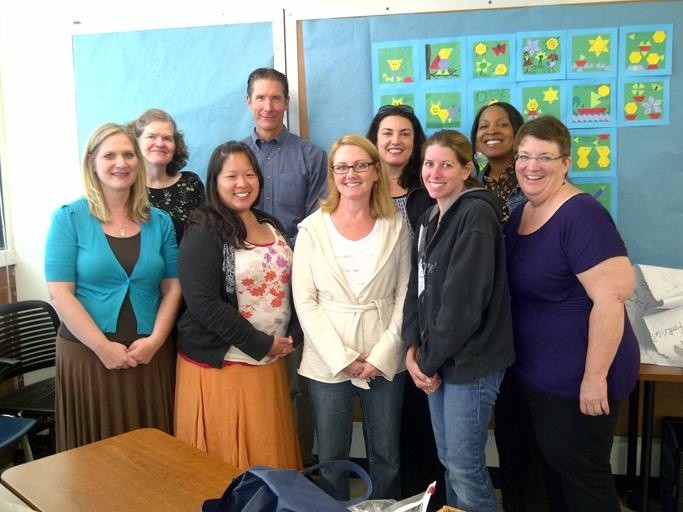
[232,68,328,248]
[501,112,644,512]
[43,119,184,456]
[398,129,518,512]
[363,102,433,236]
[167,139,307,477]
[289,135,415,505]
[463,101,525,219]
[125,109,205,253]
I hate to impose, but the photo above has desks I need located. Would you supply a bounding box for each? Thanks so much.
[0,426,246,511]
[611,365,681,512]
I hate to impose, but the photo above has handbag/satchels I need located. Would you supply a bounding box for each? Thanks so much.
[200,460,372,511]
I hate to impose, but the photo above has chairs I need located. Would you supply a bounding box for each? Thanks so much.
[0,299,60,463]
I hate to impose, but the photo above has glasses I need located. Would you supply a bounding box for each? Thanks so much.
[514,153,565,165]
[378,105,413,114]
[330,163,374,174]
